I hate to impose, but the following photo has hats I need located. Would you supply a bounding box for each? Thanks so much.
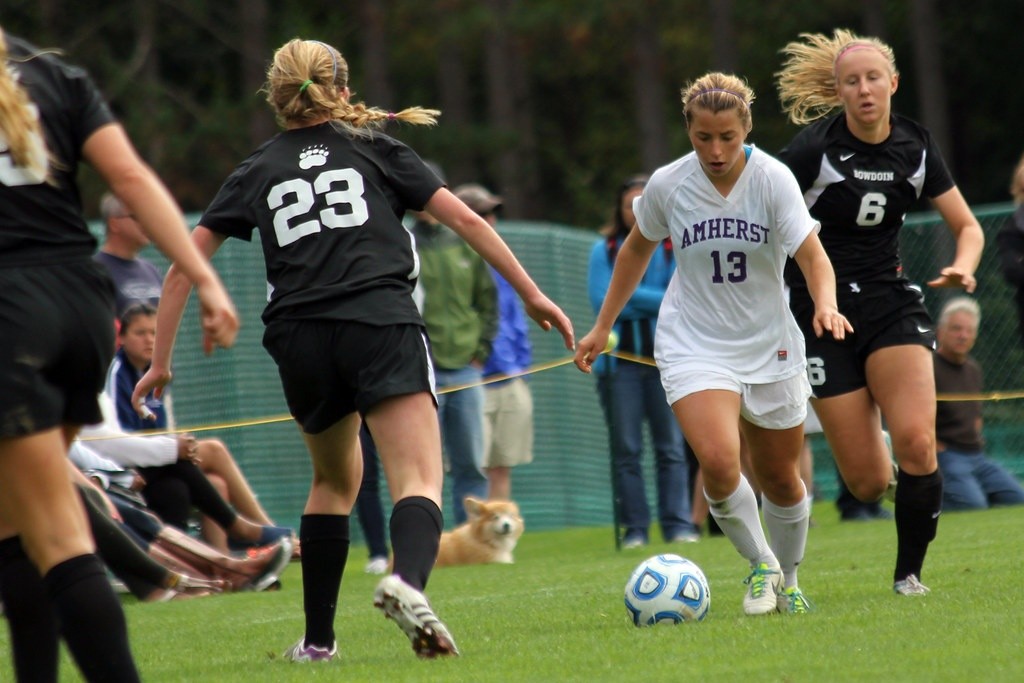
[452,182,506,216]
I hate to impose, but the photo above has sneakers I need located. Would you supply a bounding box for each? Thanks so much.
[227,525,293,551]
[893,574,931,600]
[742,562,785,616]
[776,585,816,618]
[372,573,461,662]
[281,637,342,665]
[881,430,899,489]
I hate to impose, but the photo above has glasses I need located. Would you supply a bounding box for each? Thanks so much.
[113,213,137,222]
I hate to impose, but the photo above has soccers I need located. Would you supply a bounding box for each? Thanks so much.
[624,552,712,628]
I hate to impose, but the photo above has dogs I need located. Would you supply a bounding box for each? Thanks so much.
[387,497,522,573]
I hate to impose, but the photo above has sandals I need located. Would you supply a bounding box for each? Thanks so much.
[158,573,232,601]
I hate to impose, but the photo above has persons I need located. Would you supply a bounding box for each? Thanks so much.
[776,31,986,594]
[60,161,1024,600]
[130,37,576,663]
[1,33,240,683]
[580,72,854,617]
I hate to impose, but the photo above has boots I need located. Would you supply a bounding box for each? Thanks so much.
[147,525,289,591]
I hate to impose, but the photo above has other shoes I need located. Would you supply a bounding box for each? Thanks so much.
[837,498,893,522]
[621,527,649,549]
[365,555,388,574]
[668,523,704,545]
